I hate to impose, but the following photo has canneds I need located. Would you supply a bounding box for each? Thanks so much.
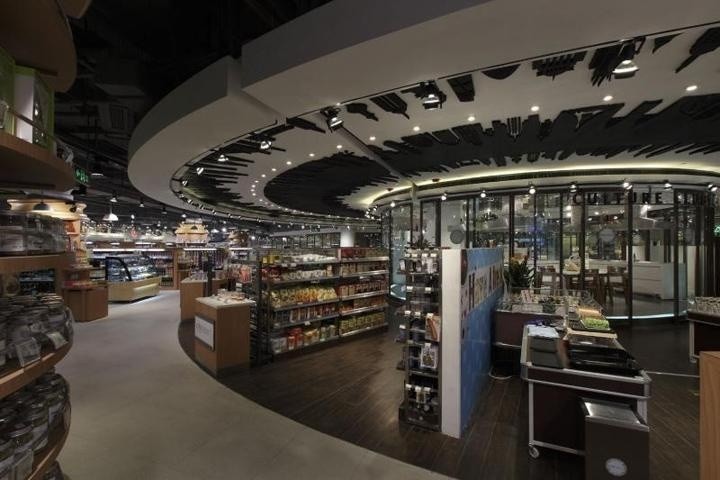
[0,290,67,480]
[0,212,66,256]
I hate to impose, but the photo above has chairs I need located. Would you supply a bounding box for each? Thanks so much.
[533,265,631,307]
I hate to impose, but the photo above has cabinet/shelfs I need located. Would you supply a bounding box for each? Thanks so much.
[396,246,442,434]
[3,211,71,480]
[698,350,720,480]
[157,225,207,284]
[489,295,606,380]
[192,296,249,371]
[686,310,720,364]
[178,250,234,315]
[102,253,160,301]
[72,265,108,321]
[248,240,388,357]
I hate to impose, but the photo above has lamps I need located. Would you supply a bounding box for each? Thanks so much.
[611,44,640,79]
[440,178,720,202]
[33,190,50,210]
[258,132,272,151]
[366,200,397,212]
[420,81,442,111]
[77,105,230,224]
[320,106,344,131]
